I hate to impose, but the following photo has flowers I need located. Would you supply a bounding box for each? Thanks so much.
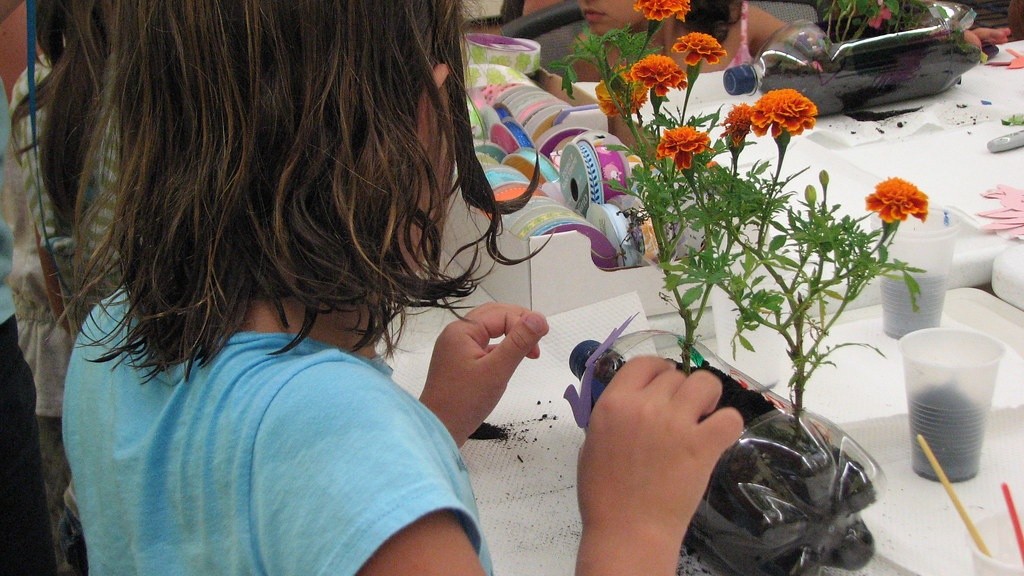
[547,0,925,446]
[813,0,958,53]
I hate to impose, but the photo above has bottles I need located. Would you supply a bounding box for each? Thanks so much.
[570,333,890,576]
[722,1,981,120]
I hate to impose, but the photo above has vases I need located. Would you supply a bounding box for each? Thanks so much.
[560,331,885,576]
[718,4,979,110]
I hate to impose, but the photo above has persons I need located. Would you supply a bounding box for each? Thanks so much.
[0,2,744,576]
[568,0,1013,82]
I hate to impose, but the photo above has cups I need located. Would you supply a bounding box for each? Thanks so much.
[898,327,1006,483]
[868,207,963,341]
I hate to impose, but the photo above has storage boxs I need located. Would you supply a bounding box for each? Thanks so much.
[408,68,728,327]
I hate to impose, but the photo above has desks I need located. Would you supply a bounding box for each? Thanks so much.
[380,39,1024,574]
